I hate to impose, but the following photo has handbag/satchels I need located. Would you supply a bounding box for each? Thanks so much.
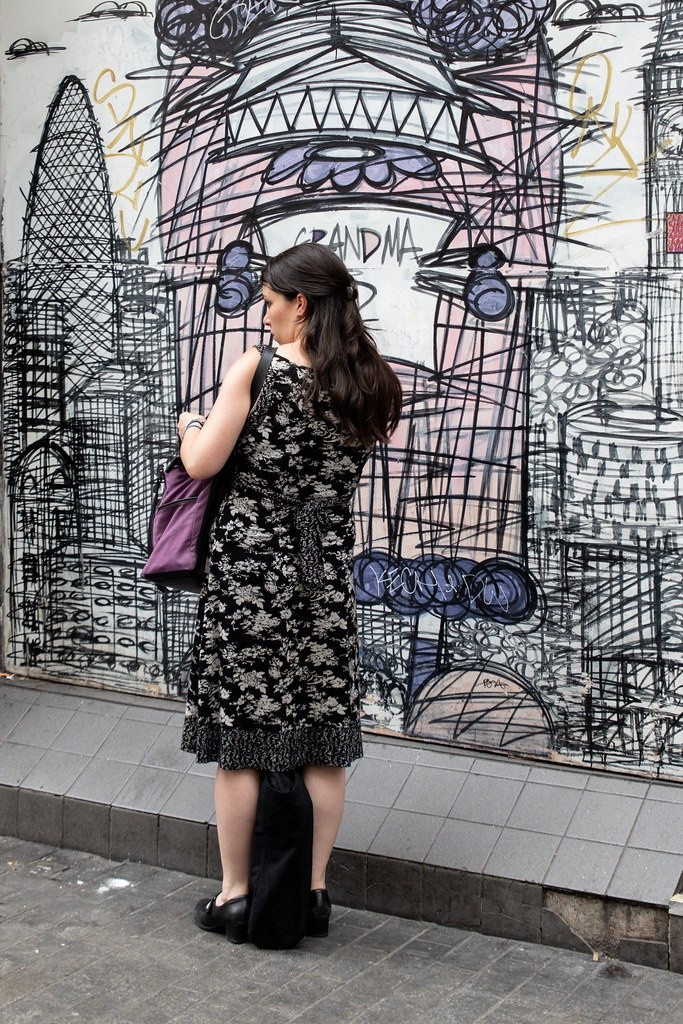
[139,448,217,593]
[246,766,313,949]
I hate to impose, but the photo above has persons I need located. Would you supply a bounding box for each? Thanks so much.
[176,240,404,948]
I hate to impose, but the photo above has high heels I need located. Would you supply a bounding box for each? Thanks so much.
[195,891,248,944]
[308,890,332,938]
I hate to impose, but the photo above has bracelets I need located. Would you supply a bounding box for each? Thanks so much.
[181,418,204,433]
